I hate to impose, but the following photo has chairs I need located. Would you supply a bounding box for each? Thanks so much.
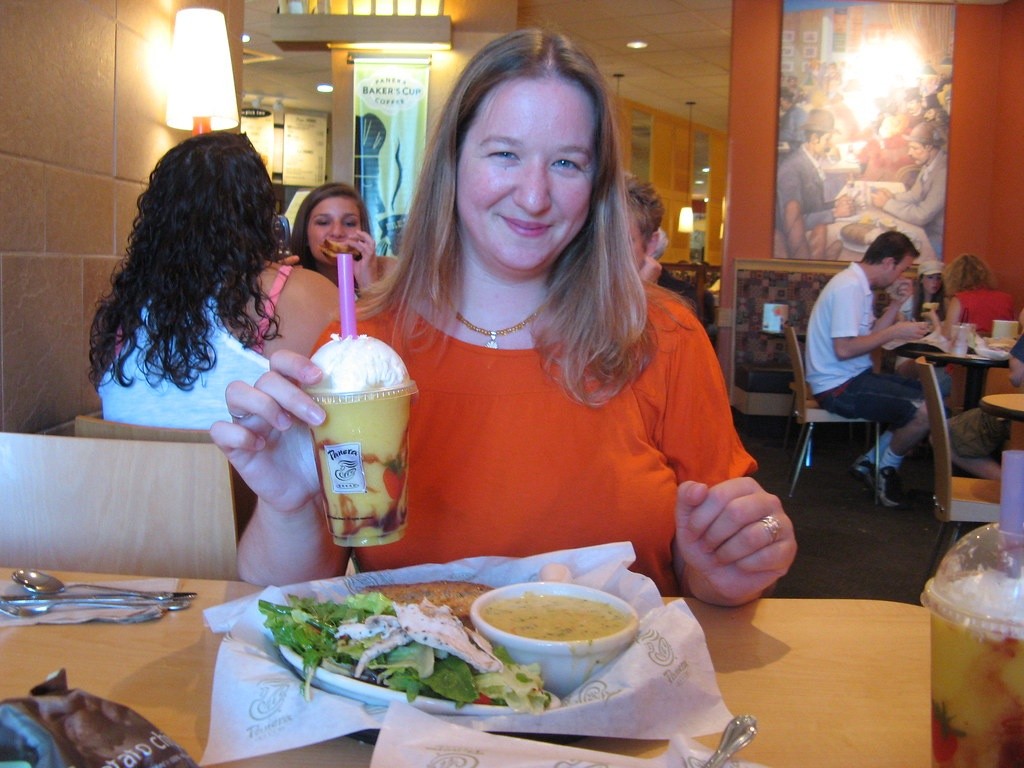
[0,413,239,580]
[914,356,1001,586]
[782,382,816,468]
[780,324,881,506]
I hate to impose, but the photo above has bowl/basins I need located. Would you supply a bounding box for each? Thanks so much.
[470,582,637,697]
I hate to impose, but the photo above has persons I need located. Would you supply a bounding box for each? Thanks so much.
[85,130,353,432]
[805,231,931,507]
[617,172,714,340]
[288,182,402,304]
[892,252,1024,481]
[210,25,798,608]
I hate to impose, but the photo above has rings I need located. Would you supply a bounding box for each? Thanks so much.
[762,516,782,541]
[229,411,251,419]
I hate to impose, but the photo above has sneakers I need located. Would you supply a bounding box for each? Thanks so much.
[849,453,907,507]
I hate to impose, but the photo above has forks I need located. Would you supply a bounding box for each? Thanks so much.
[0,602,190,617]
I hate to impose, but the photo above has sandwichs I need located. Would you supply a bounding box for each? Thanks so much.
[319,238,356,258]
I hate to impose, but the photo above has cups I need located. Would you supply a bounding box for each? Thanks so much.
[302,334,418,547]
[993,320,1018,339]
[951,325,969,355]
[921,523,1024,768]
[961,323,977,351]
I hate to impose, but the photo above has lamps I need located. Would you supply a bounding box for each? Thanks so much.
[165,6,240,136]
[677,101,696,233]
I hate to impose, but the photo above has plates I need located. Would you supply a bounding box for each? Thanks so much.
[279,642,525,716]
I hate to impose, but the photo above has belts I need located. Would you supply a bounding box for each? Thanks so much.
[814,377,857,402]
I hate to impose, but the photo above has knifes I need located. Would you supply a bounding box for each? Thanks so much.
[2,595,170,603]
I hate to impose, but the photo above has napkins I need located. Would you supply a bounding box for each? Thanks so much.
[0,577,182,629]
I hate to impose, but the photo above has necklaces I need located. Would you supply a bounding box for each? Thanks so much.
[443,292,544,349]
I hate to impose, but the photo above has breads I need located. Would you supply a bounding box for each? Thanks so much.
[362,581,498,632]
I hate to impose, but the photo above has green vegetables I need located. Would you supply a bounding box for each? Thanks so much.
[251,590,555,713]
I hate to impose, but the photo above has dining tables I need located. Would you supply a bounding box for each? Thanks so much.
[0,567,932,768]
[979,392,1024,423]
[893,345,1010,504]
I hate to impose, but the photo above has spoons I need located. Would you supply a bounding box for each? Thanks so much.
[11,569,198,600]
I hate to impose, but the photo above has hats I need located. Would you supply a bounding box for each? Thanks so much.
[918,260,944,275]
